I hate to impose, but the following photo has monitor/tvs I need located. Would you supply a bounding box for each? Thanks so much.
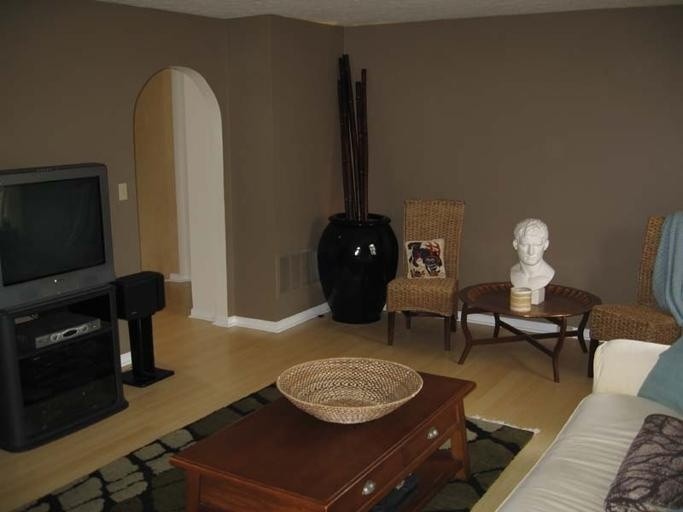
[0,162,116,311]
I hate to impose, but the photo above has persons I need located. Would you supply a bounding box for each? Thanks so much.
[506,216,558,291]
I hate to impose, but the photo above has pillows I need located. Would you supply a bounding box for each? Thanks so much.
[403,237,447,280]
[602,413,681,512]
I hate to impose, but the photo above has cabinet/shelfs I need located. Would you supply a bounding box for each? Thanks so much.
[0,288,131,453]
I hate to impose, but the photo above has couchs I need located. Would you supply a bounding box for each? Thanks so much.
[495,337,682,512]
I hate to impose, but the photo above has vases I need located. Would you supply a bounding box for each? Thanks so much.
[315,211,400,328]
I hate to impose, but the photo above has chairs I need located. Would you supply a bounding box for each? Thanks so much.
[387,197,466,351]
[587,216,683,378]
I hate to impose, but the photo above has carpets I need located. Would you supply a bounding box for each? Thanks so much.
[11,382,540,511]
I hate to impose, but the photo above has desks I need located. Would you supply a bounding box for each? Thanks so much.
[459,281,602,385]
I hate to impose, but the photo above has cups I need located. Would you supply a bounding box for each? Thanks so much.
[509,287,532,313]
